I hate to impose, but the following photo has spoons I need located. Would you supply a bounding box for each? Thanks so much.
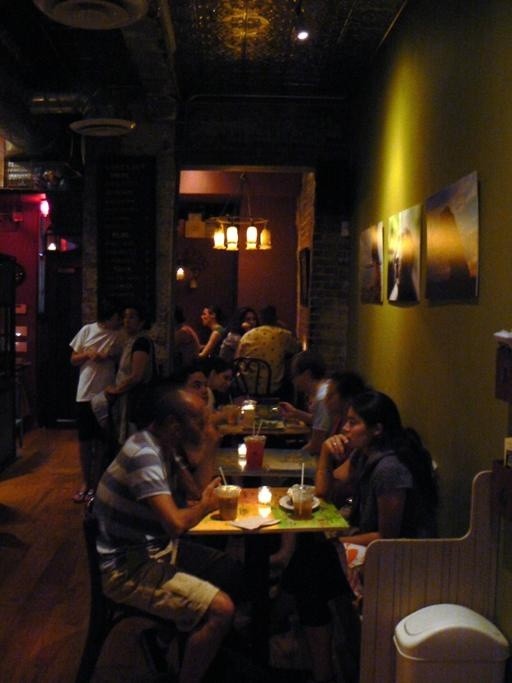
[270,404,280,416]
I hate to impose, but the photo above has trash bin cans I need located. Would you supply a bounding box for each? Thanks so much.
[392,603,511,683]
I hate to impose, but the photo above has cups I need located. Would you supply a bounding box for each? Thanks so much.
[292,485,315,520]
[242,401,256,430]
[245,436,266,469]
[238,443,247,458]
[226,405,240,431]
[214,486,240,521]
[258,506,272,520]
[256,486,272,504]
[239,459,247,471]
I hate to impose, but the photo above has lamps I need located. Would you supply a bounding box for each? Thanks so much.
[32,0,151,32]
[208,172,273,253]
[70,84,137,139]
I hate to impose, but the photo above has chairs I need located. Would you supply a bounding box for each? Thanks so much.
[230,357,272,398]
[76,493,189,682]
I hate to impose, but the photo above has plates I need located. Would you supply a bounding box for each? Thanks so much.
[255,420,278,428]
[280,494,322,510]
[287,421,305,429]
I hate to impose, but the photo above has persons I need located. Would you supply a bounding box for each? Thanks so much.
[69,301,369,569]
[91,389,236,682]
[285,389,439,683]
